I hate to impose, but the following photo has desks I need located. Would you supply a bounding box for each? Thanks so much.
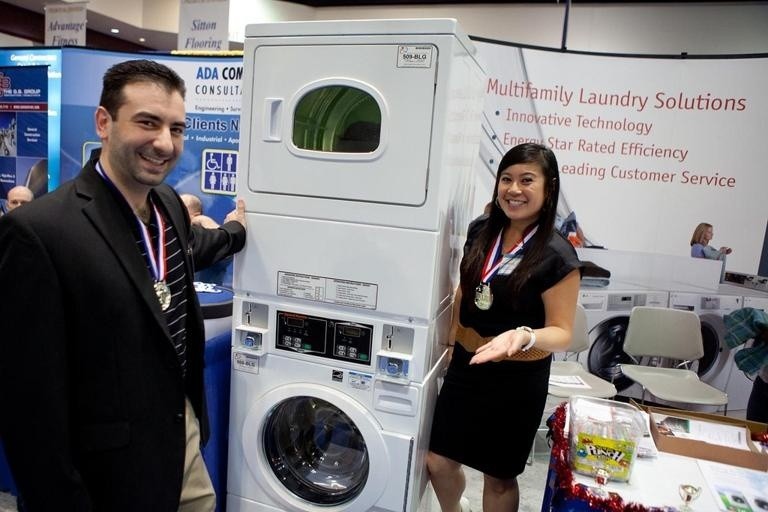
[539,401,768,512]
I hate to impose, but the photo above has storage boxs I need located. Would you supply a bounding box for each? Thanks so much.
[567,394,648,482]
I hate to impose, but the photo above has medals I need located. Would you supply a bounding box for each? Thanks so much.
[474,282,495,312]
[151,281,173,312]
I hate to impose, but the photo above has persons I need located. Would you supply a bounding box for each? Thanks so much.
[414,140,585,512]
[165,190,231,287]
[1,60,249,511]
[689,223,733,285]
[6,184,37,212]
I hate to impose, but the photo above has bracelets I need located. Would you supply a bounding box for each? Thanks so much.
[517,325,536,352]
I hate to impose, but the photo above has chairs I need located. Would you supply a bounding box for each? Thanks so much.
[525,305,730,466]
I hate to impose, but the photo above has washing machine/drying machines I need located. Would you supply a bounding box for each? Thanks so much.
[232,17,488,321]
[223,292,458,512]
